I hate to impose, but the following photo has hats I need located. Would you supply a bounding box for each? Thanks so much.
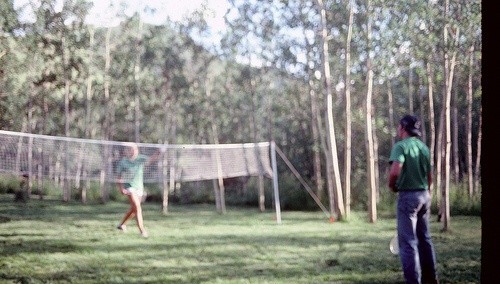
[398,115,422,136]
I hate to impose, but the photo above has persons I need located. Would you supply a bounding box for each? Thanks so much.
[115,138,169,239]
[388,116,437,284]
